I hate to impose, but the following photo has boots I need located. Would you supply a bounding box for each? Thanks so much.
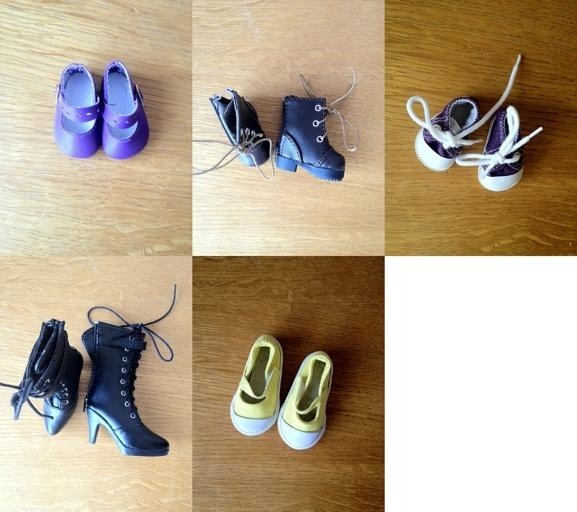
[192,87,275,182]
[82,284,176,457]
[276,67,360,182]
[0,318,83,436]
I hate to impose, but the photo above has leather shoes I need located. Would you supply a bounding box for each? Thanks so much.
[54,63,100,158]
[101,60,149,159]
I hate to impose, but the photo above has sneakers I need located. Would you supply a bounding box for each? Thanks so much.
[406,53,523,172]
[455,104,543,192]
[277,351,334,451]
[230,334,283,438]
